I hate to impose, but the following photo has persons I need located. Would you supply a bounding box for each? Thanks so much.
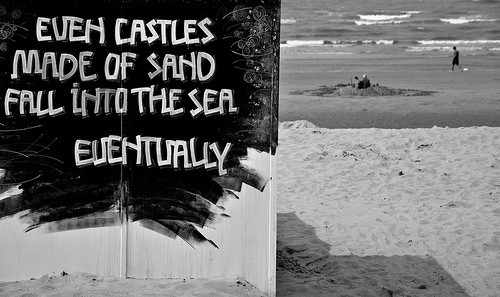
[355,73,371,90]
[448,45,464,73]
